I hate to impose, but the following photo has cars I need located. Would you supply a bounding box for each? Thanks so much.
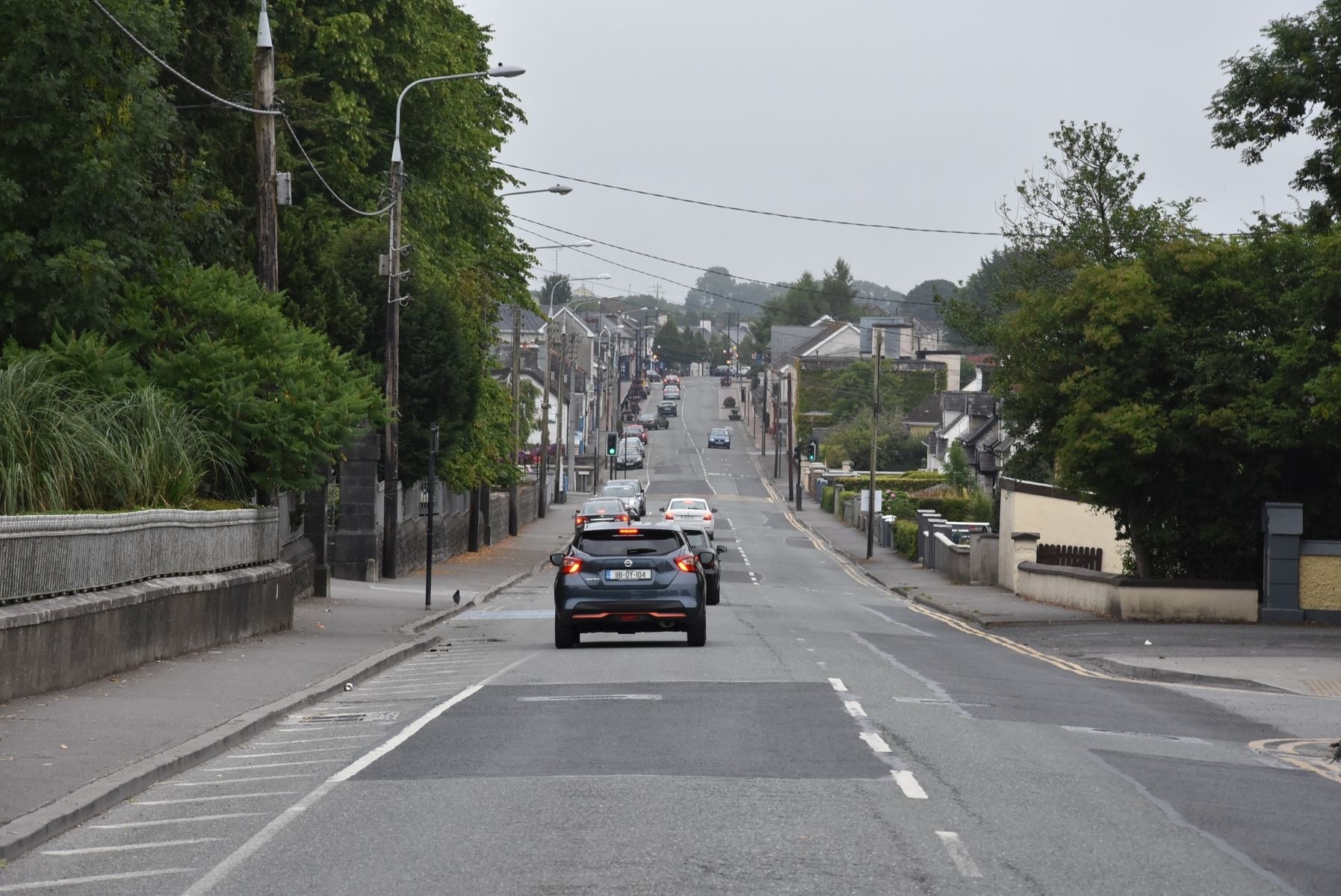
[714,366,730,377]
[617,424,648,458]
[572,500,631,537]
[663,375,680,389]
[637,413,669,430]
[680,525,728,606]
[549,498,715,647]
[575,496,631,514]
[593,485,643,522]
[728,366,751,377]
[613,448,642,470]
[663,385,681,400]
[655,400,678,417]
[659,497,719,541]
[708,427,732,449]
[645,370,662,382]
[632,379,651,395]
[606,477,646,516]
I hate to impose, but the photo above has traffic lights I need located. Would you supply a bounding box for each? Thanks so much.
[807,444,816,462]
[606,431,617,456]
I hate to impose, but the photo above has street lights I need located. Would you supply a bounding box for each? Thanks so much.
[467,184,573,552]
[380,62,526,580]
[617,324,655,414]
[595,324,625,489]
[536,272,611,518]
[616,306,649,424]
[554,294,626,504]
[507,241,594,536]
